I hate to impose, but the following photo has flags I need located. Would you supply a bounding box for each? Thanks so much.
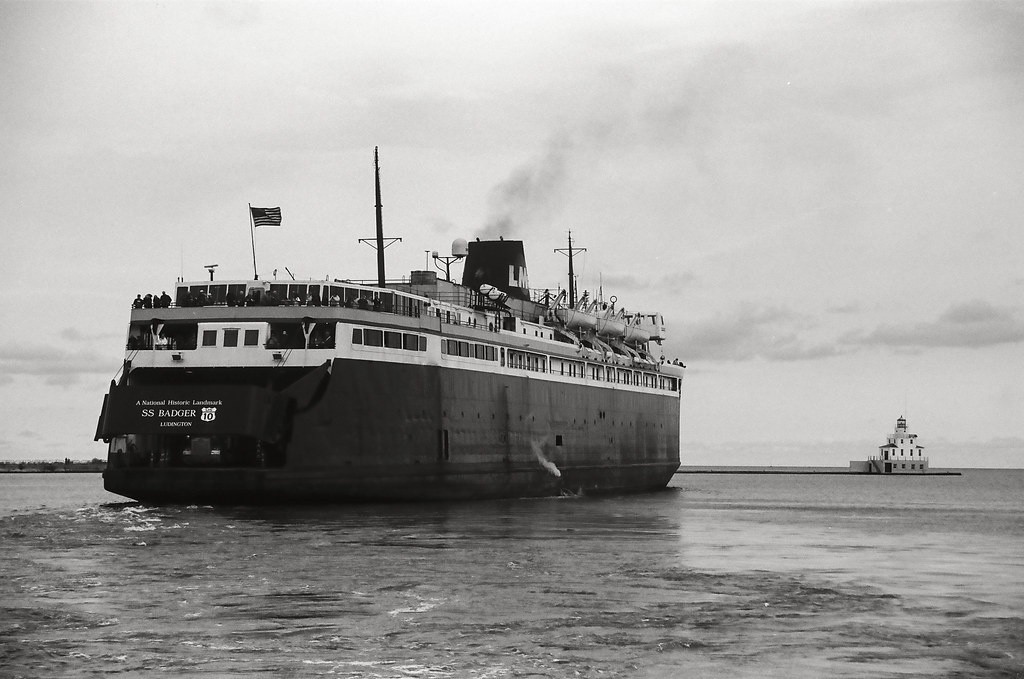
[250,206,282,227]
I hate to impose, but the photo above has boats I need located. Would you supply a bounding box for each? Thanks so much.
[94,145,686,503]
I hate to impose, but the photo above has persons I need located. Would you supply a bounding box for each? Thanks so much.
[235,290,247,307]
[226,290,237,307]
[672,358,679,365]
[371,296,385,312]
[181,290,214,307]
[245,286,258,307]
[306,294,357,308]
[153,295,161,308]
[160,291,172,308]
[357,295,370,310]
[133,294,143,309]
[142,294,153,308]
[264,290,304,306]
